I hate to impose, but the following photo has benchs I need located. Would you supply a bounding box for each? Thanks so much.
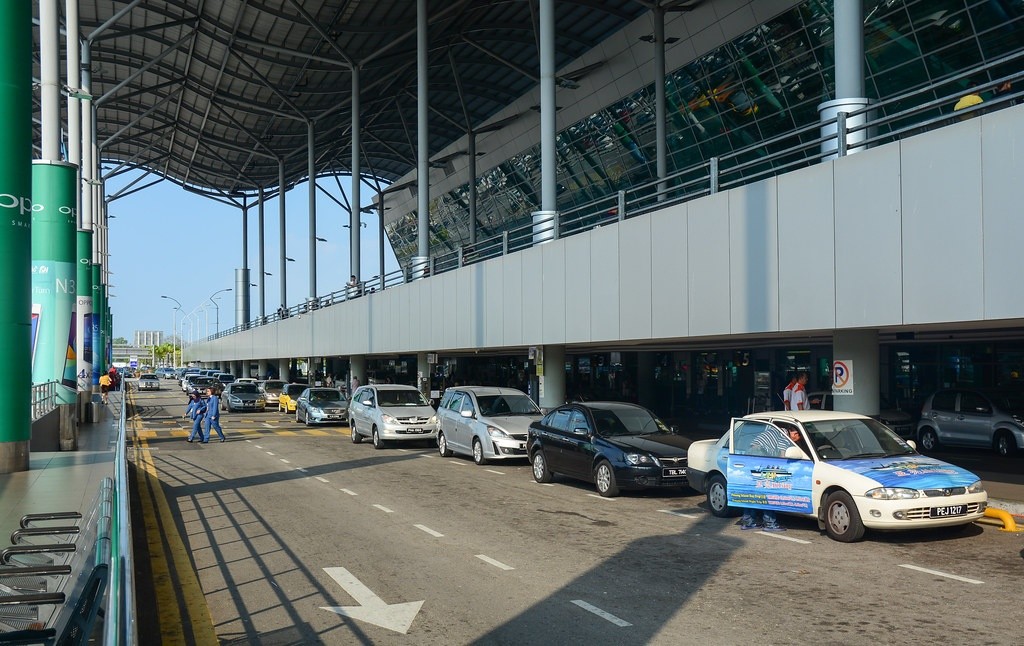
[0,478,115,646]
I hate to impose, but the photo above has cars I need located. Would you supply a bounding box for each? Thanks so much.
[221,382,266,413]
[155,367,176,380]
[173,366,290,404]
[278,383,313,415]
[685,409,989,544]
[294,387,352,426]
[137,373,160,391]
[915,387,1024,459]
[114,365,154,379]
[805,389,914,440]
[525,401,696,498]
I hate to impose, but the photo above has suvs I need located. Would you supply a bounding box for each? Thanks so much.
[349,384,437,450]
[435,386,545,465]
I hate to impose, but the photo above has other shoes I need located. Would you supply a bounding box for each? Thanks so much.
[219,436,226,442]
[187,439,193,443]
[197,439,204,442]
[201,440,208,443]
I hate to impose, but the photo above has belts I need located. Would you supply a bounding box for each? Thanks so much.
[751,444,767,454]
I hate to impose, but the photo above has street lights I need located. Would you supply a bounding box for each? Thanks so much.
[161,294,182,370]
[174,307,187,369]
[200,297,222,343]
[186,309,204,346]
[191,304,212,345]
[209,288,233,339]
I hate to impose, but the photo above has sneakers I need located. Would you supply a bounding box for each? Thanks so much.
[762,524,786,531]
[741,521,762,529]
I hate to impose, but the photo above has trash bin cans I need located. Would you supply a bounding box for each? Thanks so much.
[87,401,100,423]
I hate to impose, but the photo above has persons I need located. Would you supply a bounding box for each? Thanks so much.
[621,379,630,401]
[608,370,617,389]
[184,390,206,443]
[201,387,225,444]
[952,82,983,122]
[784,376,821,412]
[350,376,362,399]
[346,274,359,298]
[99,371,112,405]
[606,119,648,165]
[109,366,120,391]
[739,427,801,531]
[987,78,1022,114]
[385,377,394,384]
[320,371,339,388]
[479,398,491,416]
[508,370,528,394]
[698,379,706,407]
[790,372,810,412]
